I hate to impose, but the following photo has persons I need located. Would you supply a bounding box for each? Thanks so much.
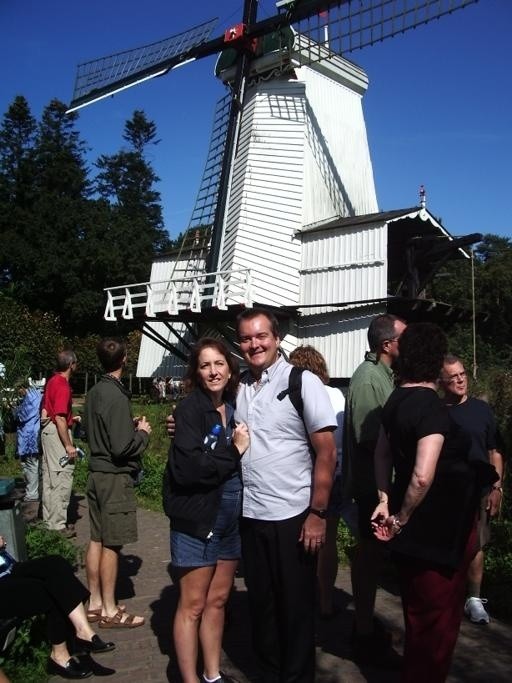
[164,308,339,683]
[287,343,348,622]
[79,337,153,630]
[377,498,387,506]
[162,336,251,683]
[340,312,408,658]
[40,350,76,540]
[152,376,181,402]
[0,533,117,680]
[370,320,482,683]
[10,374,43,503]
[436,351,503,627]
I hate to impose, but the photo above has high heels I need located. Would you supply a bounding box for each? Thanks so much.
[353,610,377,643]
[318,597,337,623]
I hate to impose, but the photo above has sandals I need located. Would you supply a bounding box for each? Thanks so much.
[89,608,105,624]
[100,609,149,628]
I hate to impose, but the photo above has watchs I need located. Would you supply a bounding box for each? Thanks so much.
[491,484,504,494]
[308,506,329,521]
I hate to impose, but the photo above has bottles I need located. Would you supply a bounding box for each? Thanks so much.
[202,423,222,450]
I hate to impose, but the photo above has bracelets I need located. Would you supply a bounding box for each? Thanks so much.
[390,512,404,537]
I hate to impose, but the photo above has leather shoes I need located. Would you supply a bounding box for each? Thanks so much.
[47,655,93,678]
[201,672,231,683]
[73,632,118,653]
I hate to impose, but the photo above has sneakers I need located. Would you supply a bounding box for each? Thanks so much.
[60,528,78,536]
[463,596,490,624]
